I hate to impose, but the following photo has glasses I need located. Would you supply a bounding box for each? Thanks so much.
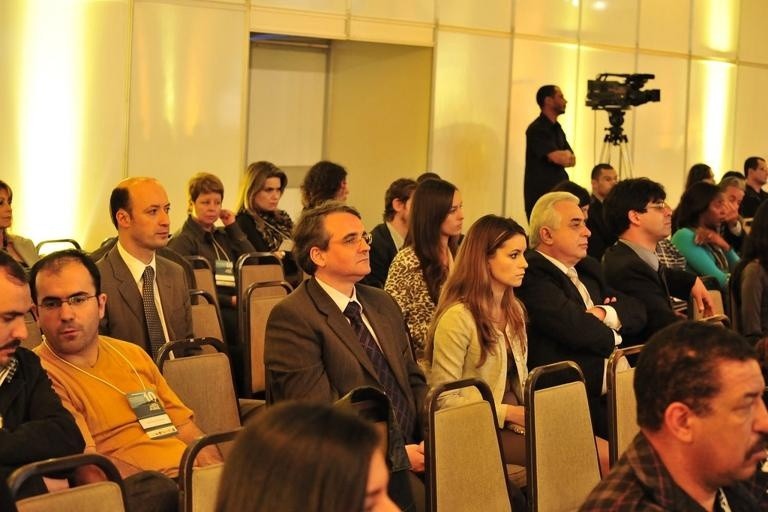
[323,232,374,247]
[641,202,668,213]
[32,294,99,312]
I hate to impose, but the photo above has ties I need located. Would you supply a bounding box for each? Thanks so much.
[655,261,674,313]
[141,264,171,376]
[564,267,596,312]
[343,300,416,445]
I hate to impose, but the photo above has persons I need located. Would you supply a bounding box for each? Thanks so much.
[524,85,576,226]
[2,157,767,510]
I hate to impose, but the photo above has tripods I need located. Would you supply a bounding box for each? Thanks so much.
[599,127,636,183]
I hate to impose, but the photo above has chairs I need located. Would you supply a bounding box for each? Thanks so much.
[188,288,227,354]
[524,361,601,511]
[688,275,726,320]
[426,377,516,512]
[155,336,244,428]
[178,427,244,512]
[185,254,218,314]
[7,453,131,512]
[606,345,646,468]
[35,238,81,257]
[701,314,733,325]
[243,281,295,400]
[235,252,287,352]
